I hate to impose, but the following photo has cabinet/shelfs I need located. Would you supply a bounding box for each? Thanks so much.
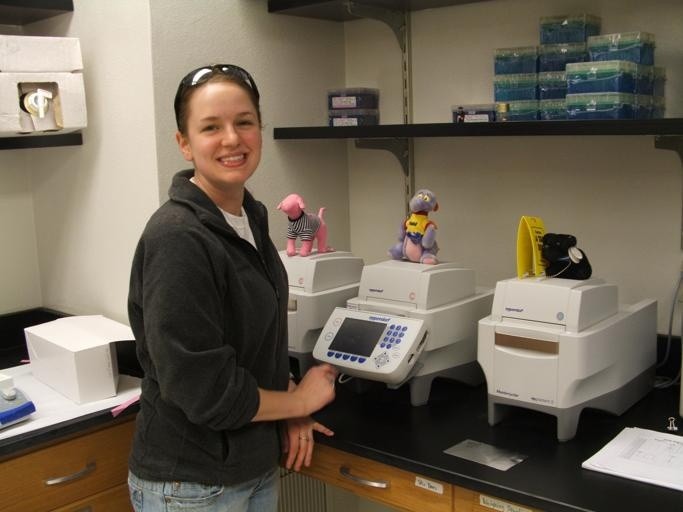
[0,0,83,149]
[268,0,683,175]
[0,402,140,512]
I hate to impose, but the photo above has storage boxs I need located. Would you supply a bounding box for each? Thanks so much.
[24,314,136,403]
[493,100,537,121]
[493,73,537,102]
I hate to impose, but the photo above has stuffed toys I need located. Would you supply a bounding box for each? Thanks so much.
[276,193,328,257]
[386,188,440,264]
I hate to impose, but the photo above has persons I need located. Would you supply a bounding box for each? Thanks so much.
[127,64,340,510]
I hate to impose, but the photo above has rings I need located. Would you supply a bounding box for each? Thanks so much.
[299,435,309,440]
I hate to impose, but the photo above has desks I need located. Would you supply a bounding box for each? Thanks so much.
[285,340,683,512]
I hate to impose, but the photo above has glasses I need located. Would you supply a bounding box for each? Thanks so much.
[181,63,252,87]
